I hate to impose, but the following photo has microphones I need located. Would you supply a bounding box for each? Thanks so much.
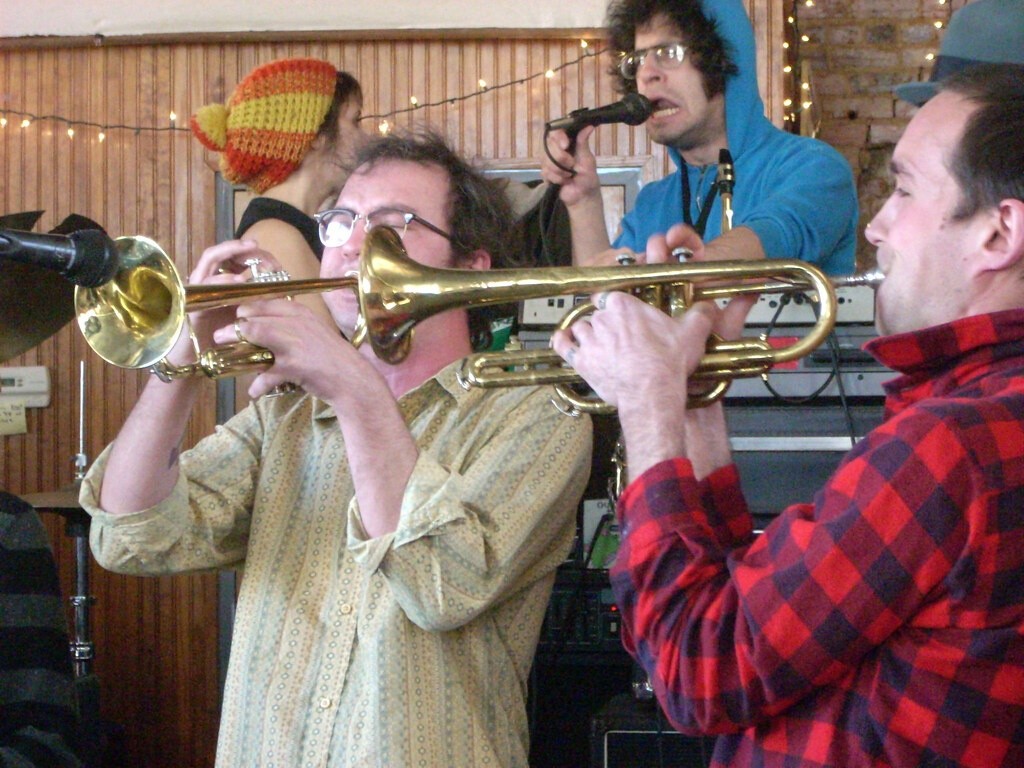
[545,93,653,132]
[0,228,118,288]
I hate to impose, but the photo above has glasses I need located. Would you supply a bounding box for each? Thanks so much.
[316,206,473,249]
[619,41,694,79]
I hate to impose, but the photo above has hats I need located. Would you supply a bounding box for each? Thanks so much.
[187,59,336,192]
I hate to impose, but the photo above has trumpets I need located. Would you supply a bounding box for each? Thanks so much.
[358,223,887,421]
[73,234,369,385]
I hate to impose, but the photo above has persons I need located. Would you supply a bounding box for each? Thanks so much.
[0,490,75,768]
[91,133,592,768]
[541,1,855,297]
[549,0,1024,767]
[190,57,366,341]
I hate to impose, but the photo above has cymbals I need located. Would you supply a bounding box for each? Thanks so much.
[0,210,109,366]
[15,489,83,510]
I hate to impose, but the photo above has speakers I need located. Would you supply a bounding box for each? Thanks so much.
[589,692,718,768]
